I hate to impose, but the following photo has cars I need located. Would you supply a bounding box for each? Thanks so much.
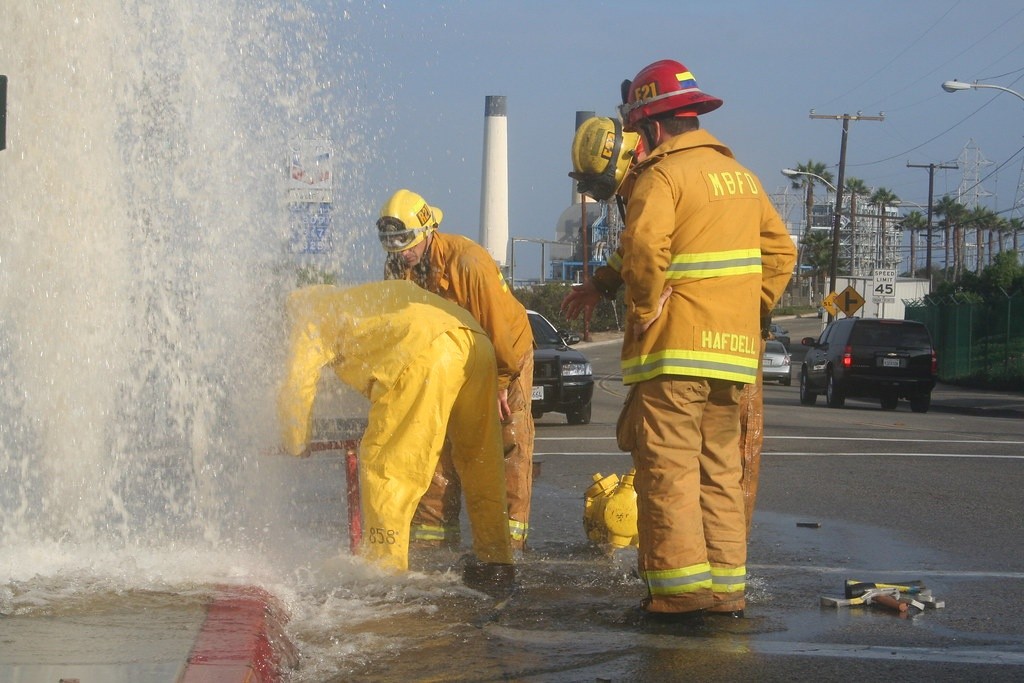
[818,306,823,317]
[765,324,791,350]
[522,309,595,426]
[763,341,792,386]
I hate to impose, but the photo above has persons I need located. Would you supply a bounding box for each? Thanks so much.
[277,279,514,585]
[376,188,535,559]
[561,59,798,621]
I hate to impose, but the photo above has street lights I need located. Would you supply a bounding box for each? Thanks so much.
[779,169,844,330]
[890,199,932,299]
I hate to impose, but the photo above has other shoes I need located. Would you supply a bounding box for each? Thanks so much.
[457,553,516,596]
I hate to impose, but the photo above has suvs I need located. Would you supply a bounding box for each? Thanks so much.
[800,316,937,410]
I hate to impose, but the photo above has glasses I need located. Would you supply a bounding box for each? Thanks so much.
[615,104,633,126]
[576,166,617,202]
[380,226,429,249]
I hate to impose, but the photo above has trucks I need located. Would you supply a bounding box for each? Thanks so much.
[822,277,931,319]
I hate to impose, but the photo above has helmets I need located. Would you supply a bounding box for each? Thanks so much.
[618,59,723,133]
[568,117,641,203]
[378,189,443,252]
